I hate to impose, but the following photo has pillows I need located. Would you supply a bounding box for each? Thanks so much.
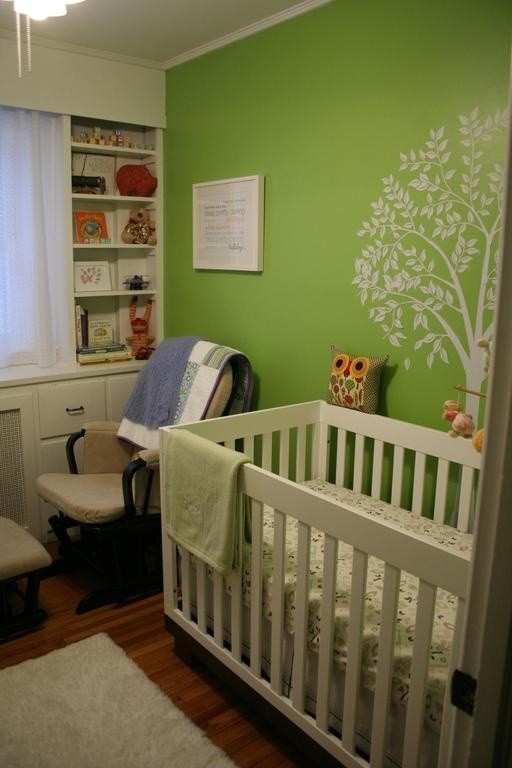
[326,345,389,415]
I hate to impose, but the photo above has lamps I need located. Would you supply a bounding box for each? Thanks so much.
[0,0,84,78]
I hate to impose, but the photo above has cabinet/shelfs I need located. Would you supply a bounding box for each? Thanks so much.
[39,115,165,365]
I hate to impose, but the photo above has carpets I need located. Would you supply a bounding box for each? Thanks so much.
[1,632,236,768]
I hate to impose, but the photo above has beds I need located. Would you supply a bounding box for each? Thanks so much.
[157,400,481,768]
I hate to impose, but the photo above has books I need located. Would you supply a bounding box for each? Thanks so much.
[76,303,132,364]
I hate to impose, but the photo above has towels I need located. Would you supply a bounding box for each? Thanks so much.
[163,426,254,573]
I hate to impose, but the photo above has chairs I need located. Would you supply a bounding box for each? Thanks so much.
[0,517,54,644]
[37,338,256,612]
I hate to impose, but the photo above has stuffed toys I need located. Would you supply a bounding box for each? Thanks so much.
[125,295,155,355]
[469,429,485,453]
[442,400,475,439]
[121,207,156,245]
